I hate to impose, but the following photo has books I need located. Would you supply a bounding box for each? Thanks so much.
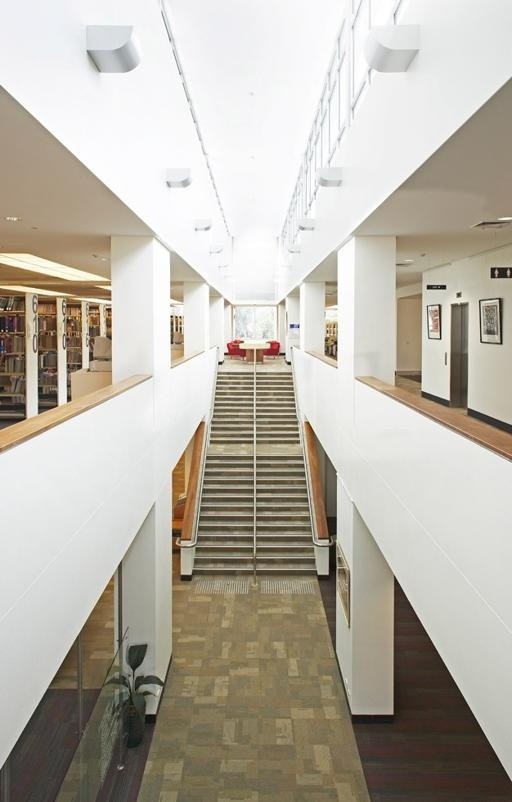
[0,294,184,412]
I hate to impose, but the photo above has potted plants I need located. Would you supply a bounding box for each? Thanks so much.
[102,643,166,747]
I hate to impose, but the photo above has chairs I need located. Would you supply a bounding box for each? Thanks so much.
[227,340,280,360]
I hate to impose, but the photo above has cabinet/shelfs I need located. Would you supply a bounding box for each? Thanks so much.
[0,295,112,420]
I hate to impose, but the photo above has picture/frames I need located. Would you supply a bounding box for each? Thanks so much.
[426,304,441,340]
[335,540,350,628]
[479,298,502,345]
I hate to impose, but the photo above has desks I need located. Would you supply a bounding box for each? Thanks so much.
[239,339,270,364]
